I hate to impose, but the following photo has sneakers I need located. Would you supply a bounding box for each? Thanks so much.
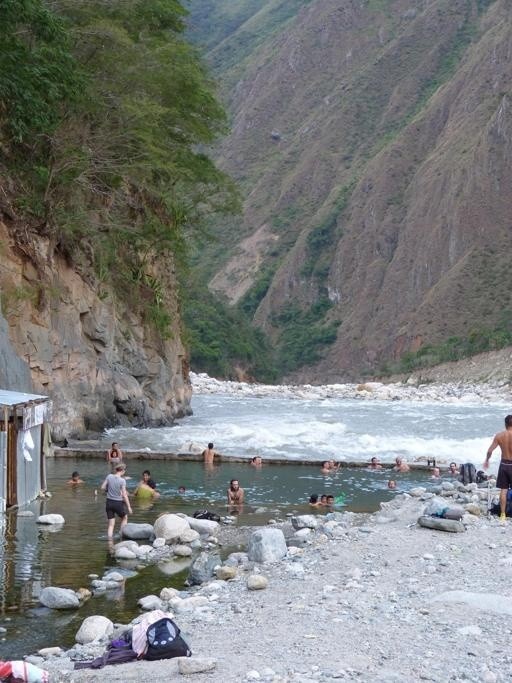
[500,513,506,520]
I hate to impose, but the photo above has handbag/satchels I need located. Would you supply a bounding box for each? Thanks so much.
[146,618,191,660]
[476,471,487,483]
[194,509,210,519]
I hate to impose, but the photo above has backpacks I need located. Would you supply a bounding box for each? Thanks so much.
[459,463,476,486]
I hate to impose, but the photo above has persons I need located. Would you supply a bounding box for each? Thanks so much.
[431,468,441,479]
[482,414,512,520]
[387,479,397,488]
[308,493,334,506]
[328,459,338,469]
[227,478,245,504]
[393,456,410,472]
[201,442,221,463]
[178,485,186,494]
[448,462,459,474]
[250,456,262,464]
[320,460,329,474]
[367,457,383,468]
[67,442,161,547]
